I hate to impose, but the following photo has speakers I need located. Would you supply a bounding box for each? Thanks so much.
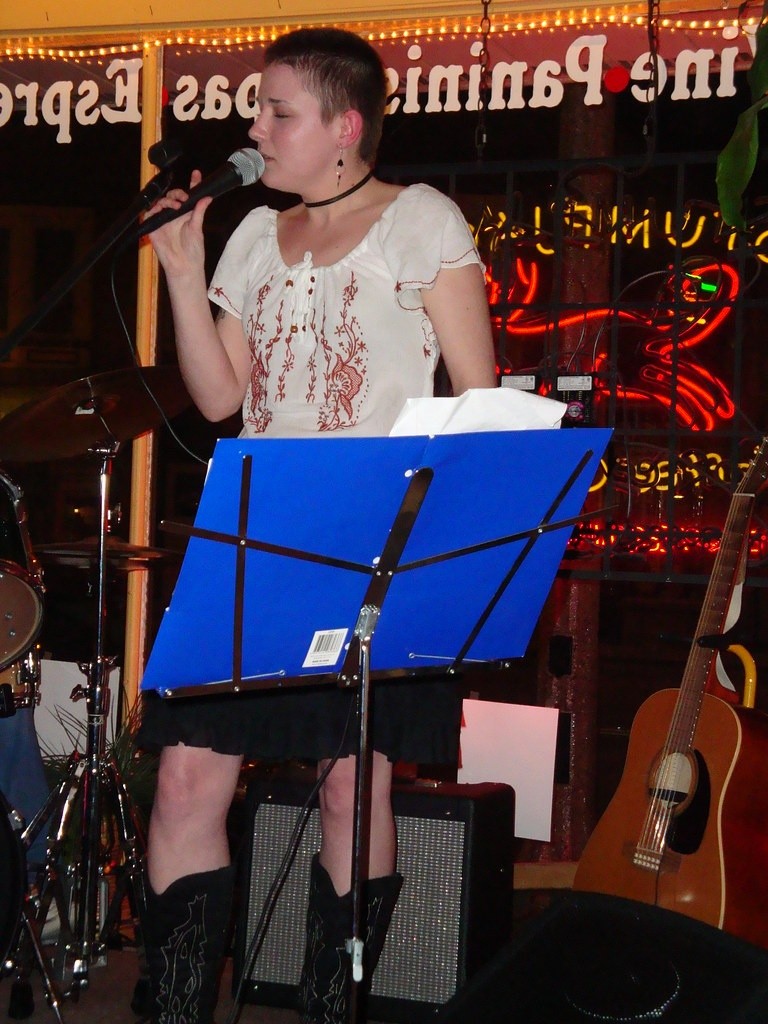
[232,779,515,1024]
[425,888,768,1024]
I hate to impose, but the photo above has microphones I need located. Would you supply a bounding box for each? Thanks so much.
[133,148,264,235]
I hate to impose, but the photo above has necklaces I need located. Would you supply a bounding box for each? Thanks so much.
[305,167,373,209]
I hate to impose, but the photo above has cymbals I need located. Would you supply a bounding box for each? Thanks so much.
[1,367,193,465]
[32,536,186,572]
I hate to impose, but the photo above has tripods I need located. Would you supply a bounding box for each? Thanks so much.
[22,439,159,1005]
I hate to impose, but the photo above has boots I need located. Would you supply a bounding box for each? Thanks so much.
[300,852,405,1024]
[135,857,239,1024]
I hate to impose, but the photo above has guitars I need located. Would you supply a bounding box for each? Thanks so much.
[572,436,767,938]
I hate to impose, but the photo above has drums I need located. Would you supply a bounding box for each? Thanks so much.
[0,466,38,668]
[0,648,44,710]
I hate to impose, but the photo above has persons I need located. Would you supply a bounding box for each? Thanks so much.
[144,26,503,1024]
